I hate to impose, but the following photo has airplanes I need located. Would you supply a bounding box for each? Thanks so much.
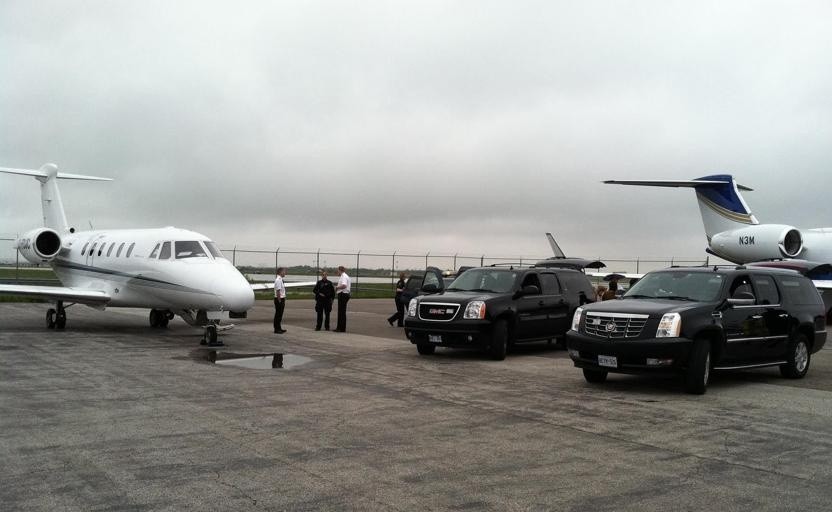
[533,231,606,271]
[208,351,285,370]
[0,162,318,345]
[601,173,832,282]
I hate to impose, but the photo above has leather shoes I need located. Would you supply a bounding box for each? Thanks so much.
[333,328,347,332]
[281,329,287,333]
[274,330,283,334]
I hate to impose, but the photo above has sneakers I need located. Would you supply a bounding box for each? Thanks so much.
[325,327,330,331]
[387,318,394,327]
[314,327,321,331]
[398,325,406,328]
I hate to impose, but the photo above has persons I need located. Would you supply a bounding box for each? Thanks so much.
[312,272,335,331]
[387,272,408,327]
[332,265,351,332]
[592,277,624,302]
[273,267,287,334]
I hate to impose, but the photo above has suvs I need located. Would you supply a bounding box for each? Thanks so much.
[405,268,598,361]
[404,269,459,311]
[566,266,826,395]
